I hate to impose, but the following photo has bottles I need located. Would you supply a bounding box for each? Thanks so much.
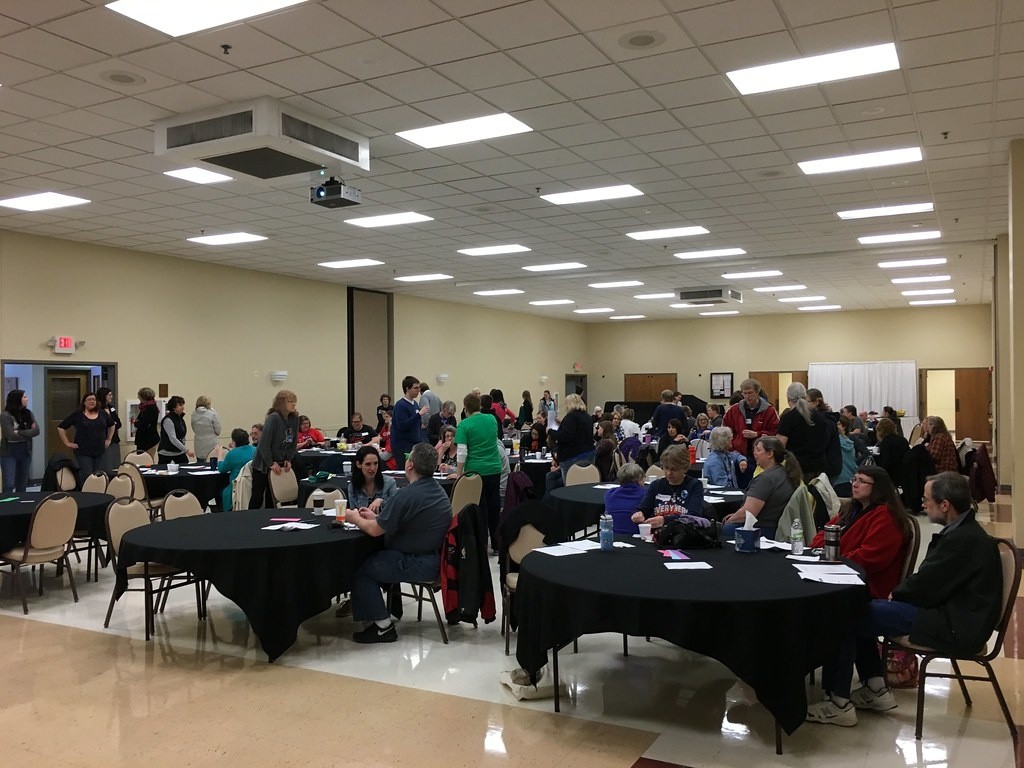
[689,446,696,464]
[340,434,345,450]
[792,518,804,554]
[600,511,613,552]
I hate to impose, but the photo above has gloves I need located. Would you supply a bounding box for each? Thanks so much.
[680,514,710,528]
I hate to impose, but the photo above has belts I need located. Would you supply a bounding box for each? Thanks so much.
[408,550,442,556]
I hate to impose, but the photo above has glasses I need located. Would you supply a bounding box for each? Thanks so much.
[352,420,363,425]
[412,386,420,391]
[250,431,260,436]
[612,417,620,421]
[849,477,874,486]
[921,496,941,505]
[741,391,757,396]
[447,411,454,416]
[641,475,646,481]
[86,398,96,401]
[698,418,708,421]
[284,400,296,404]
[531,432,539,437]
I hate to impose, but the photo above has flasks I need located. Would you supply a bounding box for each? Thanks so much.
[825,525,840,560]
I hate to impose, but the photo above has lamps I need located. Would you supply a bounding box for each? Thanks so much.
[436,373,449,383]
[272,371,287,380]
[46,336,86,354]
[539,375,548,383]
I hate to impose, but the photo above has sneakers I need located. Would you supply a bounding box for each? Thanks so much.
[336,599,352,617]
[805,699,859,726]
[387,607,403,621]
[353,621,398,644]
[850,684,899,710]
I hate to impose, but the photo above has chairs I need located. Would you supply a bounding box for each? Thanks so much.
[0,425,1024,740]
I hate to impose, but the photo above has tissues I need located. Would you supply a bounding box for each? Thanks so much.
[734,509,760,554]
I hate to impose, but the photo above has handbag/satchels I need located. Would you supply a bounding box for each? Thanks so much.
[653,517,719,547]
[876,641,920,688]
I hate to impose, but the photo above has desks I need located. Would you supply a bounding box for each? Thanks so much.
[0,491,117,593]
[507,537,873,755]
[97,461,231,514]
[298,470,457,508]
[295,446,356,486]
[507,452,553,494]
[545,482,747,544]
[112,508,386,664]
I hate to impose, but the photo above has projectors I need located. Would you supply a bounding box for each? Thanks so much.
[309,185,362,209]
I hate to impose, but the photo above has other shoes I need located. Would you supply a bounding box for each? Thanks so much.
[493,550,497,556]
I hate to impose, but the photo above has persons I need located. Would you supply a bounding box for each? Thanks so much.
[214,428,258,512]
[335,445,397,616]
[0,389,40,492]
[446,393,503,556]
[809,465,912,600]
[251,424,264,448]
[631,444,706,535]
[156,396,196,464]
[604,464,648,537]
[345,443,453,644]
[98,388,124,481]
[134,388,160,462]
[248,391,300,510]
[191,396,221,463]
[296,416,326,477]
[57,393,115,490]
[369,375,963,539]
[804,470,1003,725]
[341,412,378,445]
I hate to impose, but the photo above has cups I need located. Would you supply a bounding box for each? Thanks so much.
[211,458,217,470]
[347,443,359,450]
[535,452,541,459]
[312,492,325,515]
[699,478,708,489]
[638,524,652,540]
[542,447,547,455]
[335,500,347,521]
[167,464,179,472]
[649,476,657,483]
[343,462,352,476]
[506,449,511,456]
[372,444,377,449]
[325,439,330,450]
[873,447,878,453]
[646,434,651,444]
